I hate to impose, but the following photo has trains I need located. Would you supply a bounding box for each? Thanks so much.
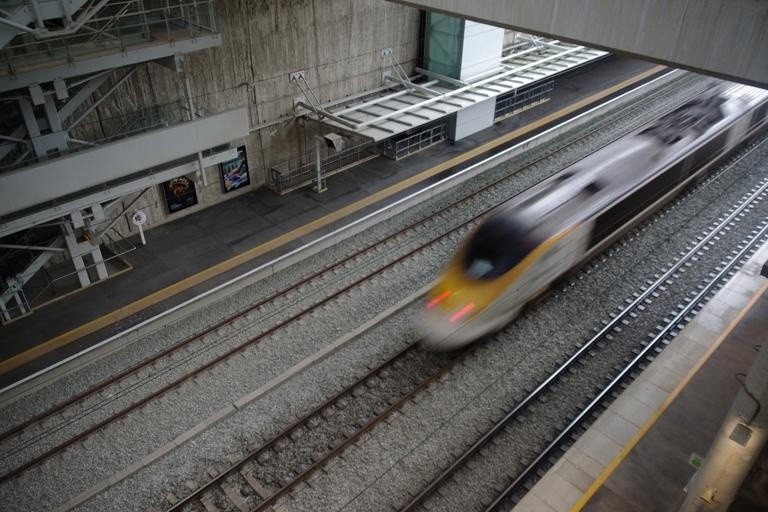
[412,81,768,354]
